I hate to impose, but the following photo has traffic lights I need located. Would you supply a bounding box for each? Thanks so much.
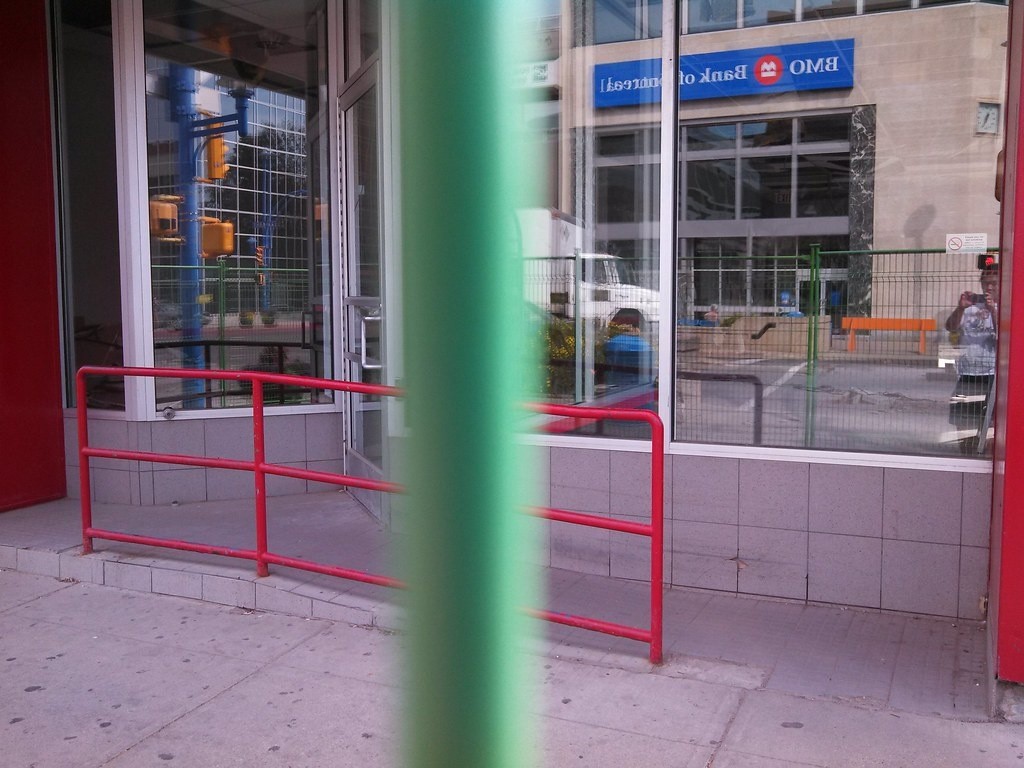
[255,246,265,266]
[197,108,231,183]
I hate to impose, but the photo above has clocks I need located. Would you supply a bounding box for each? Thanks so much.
[976,102,1002,135]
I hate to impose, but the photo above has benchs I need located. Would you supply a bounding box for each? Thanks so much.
[841,317,937,355]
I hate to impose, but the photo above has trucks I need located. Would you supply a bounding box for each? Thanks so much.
[519,207,660,337]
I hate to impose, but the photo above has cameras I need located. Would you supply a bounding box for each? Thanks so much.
[970,293,988,303]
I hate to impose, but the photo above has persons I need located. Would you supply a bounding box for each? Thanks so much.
[777,292,796,307]
[704,303,718,322]
[944,264,1000,454]
